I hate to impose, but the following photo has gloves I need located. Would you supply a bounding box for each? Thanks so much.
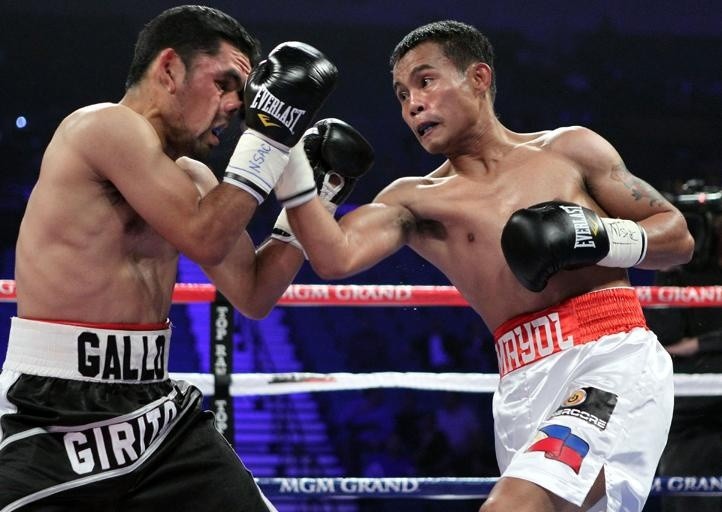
[501,200,648,292]
[222,40,378,261]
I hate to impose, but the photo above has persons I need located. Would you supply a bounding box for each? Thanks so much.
[655,182,721,374]
[0,4,374,512]
[237,21,694,512]
